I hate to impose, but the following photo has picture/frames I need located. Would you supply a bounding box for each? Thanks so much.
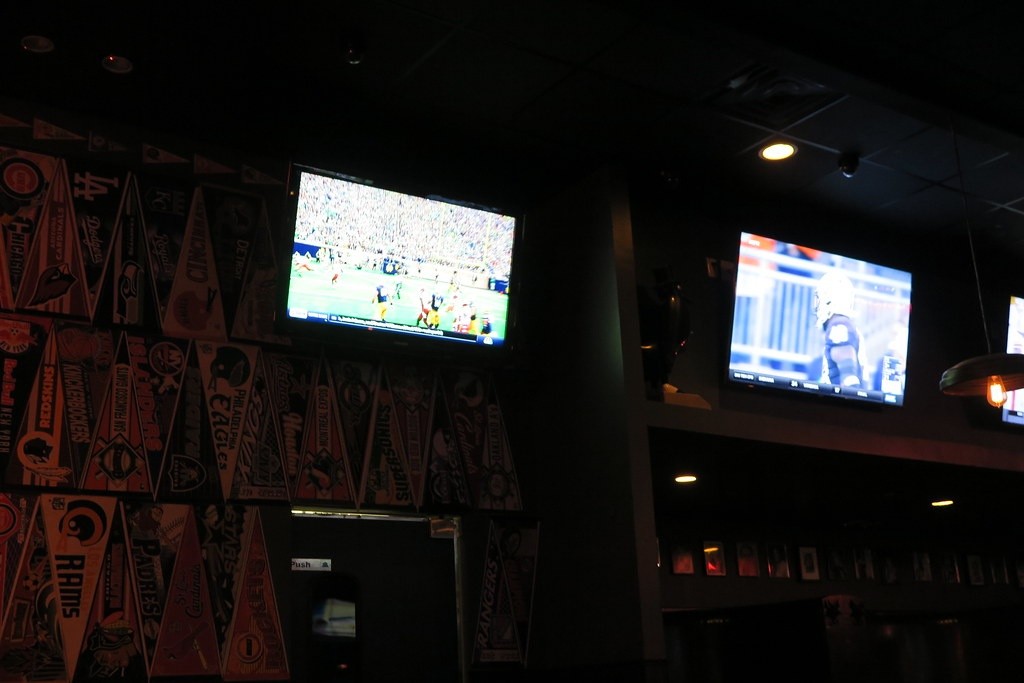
[670,540,1023,590]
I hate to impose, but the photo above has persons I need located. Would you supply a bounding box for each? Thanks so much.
[812,272,871,390]
[739,543,759,573]
[768,543,789,576]
[293,172,514,337]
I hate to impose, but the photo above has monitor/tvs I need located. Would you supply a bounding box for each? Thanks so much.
[272,161,526,364]
[720,228,919,416]
[997,295,1024,426]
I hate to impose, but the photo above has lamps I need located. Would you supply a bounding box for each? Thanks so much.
[938,120,1024,407]
